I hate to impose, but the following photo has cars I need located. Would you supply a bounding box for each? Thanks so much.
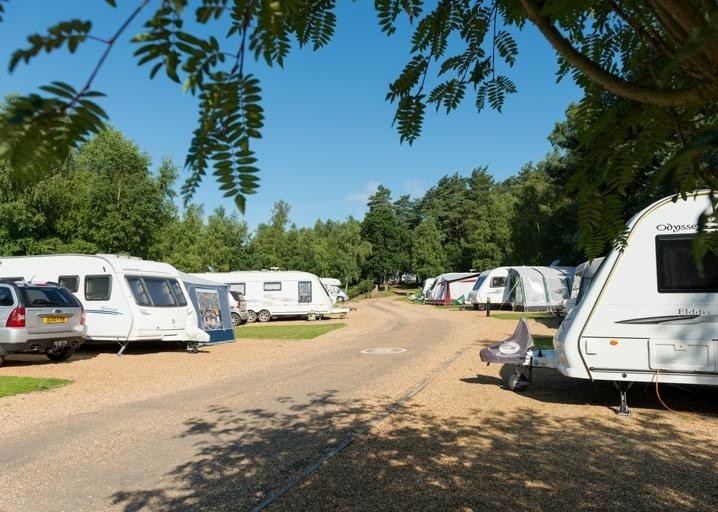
[1,277,87,361]
[194,291,248,326]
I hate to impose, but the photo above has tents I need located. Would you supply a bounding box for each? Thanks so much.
[178,273,234,345]
[426,273,480,304]
[503,267,576,312]
